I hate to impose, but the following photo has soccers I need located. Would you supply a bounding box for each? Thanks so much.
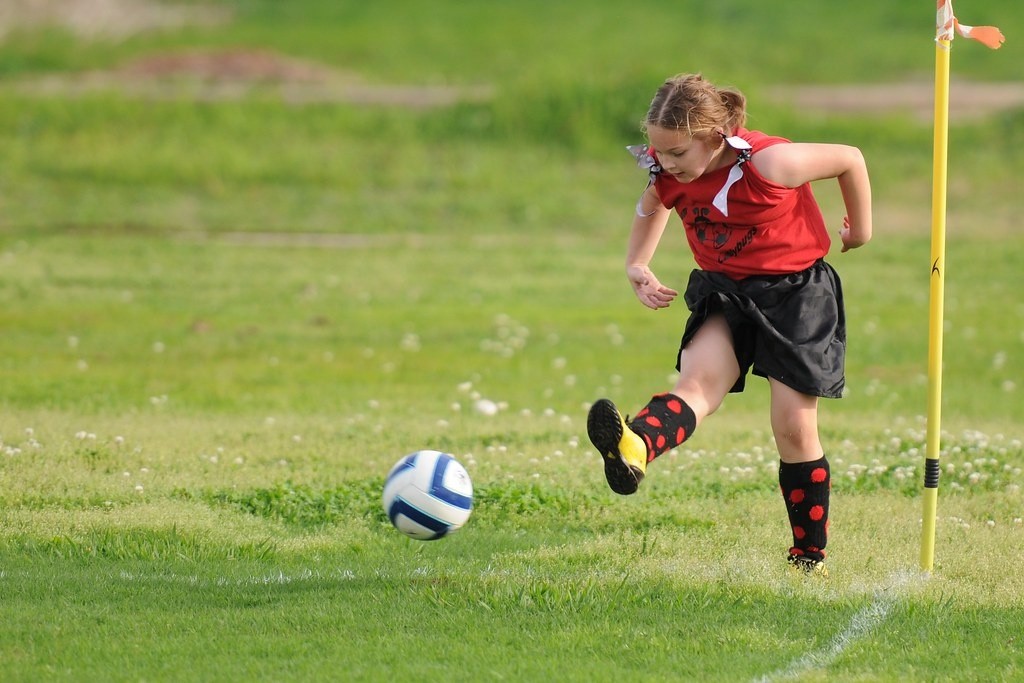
[381,449,474,542]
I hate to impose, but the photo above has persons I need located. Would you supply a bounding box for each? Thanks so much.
[587,73,874,576]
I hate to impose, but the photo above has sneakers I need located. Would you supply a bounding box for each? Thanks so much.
[786,555,831,582]
[586,398,650,496]
